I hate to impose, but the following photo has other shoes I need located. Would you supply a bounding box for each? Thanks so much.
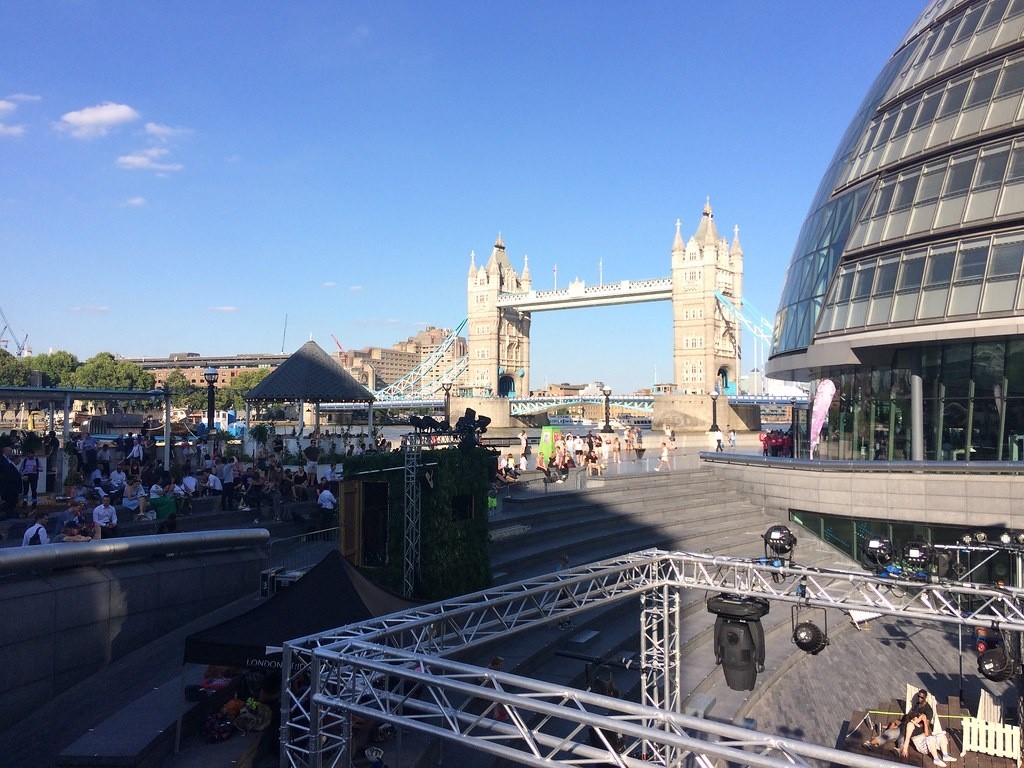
[653,467,659,471]
[242,507,250,511]
[179,512,184,517]
[187,511,192,515]
[556,480,564,483]
[863,741,872,750]
[943,757,957,762]
[252,519,259,524]
[238,506,244,509]
[893,748,901,757]
[933,760,946,767]
[138,513,148,521]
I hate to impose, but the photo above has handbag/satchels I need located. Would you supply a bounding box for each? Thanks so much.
[721,443,724,449]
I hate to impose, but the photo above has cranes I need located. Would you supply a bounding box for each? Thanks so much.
[0,308,29,357]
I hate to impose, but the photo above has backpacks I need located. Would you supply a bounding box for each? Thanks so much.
[137,445,148,467]
[29,527,42,545]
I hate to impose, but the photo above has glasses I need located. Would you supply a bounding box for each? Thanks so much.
[918,695,924,699]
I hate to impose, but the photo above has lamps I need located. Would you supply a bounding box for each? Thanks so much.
[977,647,1020,681]
[901,535,937,576]
[410,407,491,447]
[960,531,1024,550]
[950,563,965,579]
[762,524,797,565]
[863,536,894,577]
[791,604,830,656]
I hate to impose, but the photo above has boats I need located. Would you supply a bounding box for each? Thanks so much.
[547,413,652,430]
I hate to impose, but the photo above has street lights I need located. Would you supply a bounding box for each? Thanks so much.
[601,386,615,433]
[441,378,454,424]
[708,391,721,432]
[202,367,220,429]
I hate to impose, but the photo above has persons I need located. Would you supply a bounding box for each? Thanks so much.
[762,429,794,458]
[862,689,957,768]
[494,424,737,484]
[0,416,392,547]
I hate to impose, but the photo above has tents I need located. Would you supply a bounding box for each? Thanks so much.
[176,553,448,756]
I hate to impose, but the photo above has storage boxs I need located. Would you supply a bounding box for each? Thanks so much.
[259,565,285,597]
[275,569,307,595]
